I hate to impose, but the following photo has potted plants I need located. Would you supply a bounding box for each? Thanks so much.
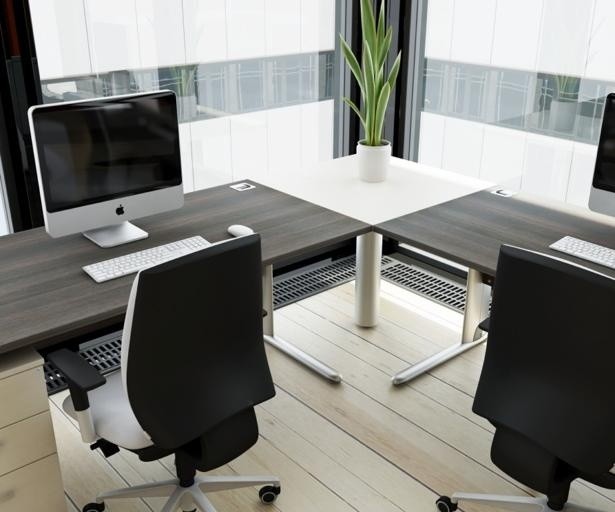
[338,1,402,182]
[548,74,581,133]
[170,64,199,122]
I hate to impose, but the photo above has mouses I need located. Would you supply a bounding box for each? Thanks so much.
[227,224,254,237]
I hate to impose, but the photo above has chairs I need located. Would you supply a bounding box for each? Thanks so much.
[45,233,282,512]
[435,243,615,512]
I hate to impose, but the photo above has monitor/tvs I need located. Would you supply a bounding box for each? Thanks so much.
[27,90,186,249]
[588,93,615,216]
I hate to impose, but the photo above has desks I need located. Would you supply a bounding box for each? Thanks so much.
[372,185,615,387]
[245,153,494,329]
[1,150,372,511]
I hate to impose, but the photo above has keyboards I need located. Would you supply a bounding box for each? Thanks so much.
[81,235,212,284]
[549,235,615,270]
